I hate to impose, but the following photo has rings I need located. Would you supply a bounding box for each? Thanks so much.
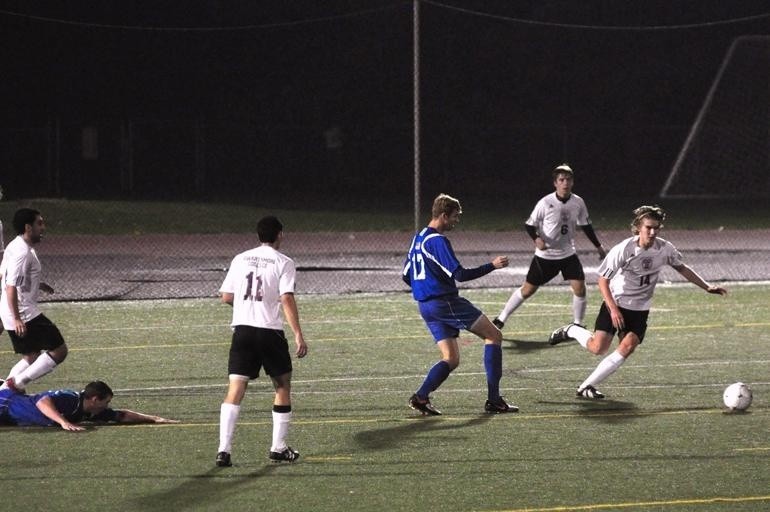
[21,328,24,332]
[617,319,620,322]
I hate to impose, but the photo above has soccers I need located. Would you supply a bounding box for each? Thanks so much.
[723,382,752,410]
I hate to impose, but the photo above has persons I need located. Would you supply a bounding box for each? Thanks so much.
[0,380,180,431]
[216,216,308,467]
[0,220,5,336]
[548,205,727,398]
[493,163,607,331]
[402,193,519,415]
[0,208,67,395]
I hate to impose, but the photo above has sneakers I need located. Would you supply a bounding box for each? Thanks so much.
[485,396,519,414]
[576,385,605,398]
[270,446,299,464]
[409,394,442,415]
[216,452,232,467]
[6,377,26,394]
[549,322,577,346]
